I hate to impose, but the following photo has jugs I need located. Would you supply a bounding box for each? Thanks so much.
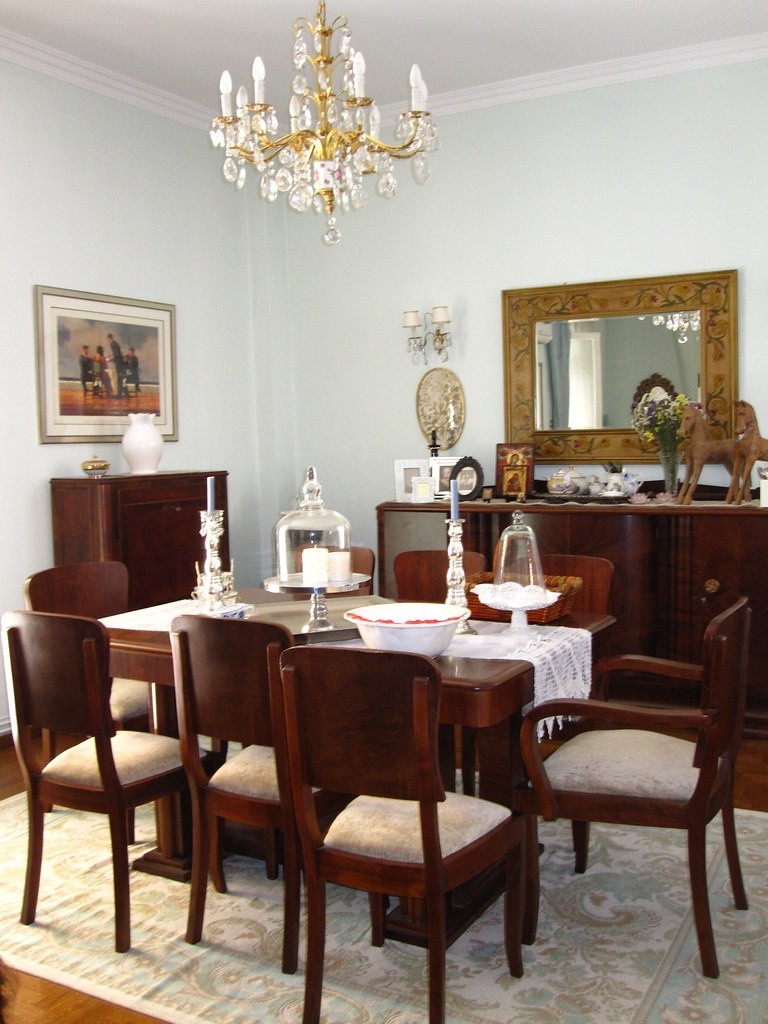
[543,466,644,497]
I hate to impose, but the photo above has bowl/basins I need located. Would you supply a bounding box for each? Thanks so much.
[343,602,472,660]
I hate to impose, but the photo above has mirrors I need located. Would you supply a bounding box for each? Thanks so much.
[501,269,737,460]
[631,372,681,420]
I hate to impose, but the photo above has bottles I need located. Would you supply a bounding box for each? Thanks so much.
[121,412,164,474]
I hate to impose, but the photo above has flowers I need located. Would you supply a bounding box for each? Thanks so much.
[632,393,701,498]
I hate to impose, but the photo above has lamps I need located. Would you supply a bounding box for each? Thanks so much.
[210,0,441,245]
[638,310,702,345]
[402,305,452,368]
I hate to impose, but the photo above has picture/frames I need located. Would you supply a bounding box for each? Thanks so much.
[412,477,434,502]
[35,284,180,443]
[450,456,484,501]
[431,457,466,497]
[395,458,429,502]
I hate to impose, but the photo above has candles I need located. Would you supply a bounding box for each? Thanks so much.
[328,551,351,582]
[207,475,216,513]
[302,543,329,587]
[450,478,458,521]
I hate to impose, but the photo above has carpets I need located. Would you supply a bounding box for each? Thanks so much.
[0,727,768,1024]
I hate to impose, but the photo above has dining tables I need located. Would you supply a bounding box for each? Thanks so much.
[86,588,616,952]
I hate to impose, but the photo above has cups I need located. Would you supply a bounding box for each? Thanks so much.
[218,603,255,621]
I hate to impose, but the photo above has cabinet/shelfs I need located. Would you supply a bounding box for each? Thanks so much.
[375,492,768,738]
[48,470,232,612]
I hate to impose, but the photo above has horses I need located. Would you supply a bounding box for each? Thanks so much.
[676,405,753,505]
[726,400,768,505]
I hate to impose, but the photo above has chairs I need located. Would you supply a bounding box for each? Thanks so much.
[2,535,748,1024]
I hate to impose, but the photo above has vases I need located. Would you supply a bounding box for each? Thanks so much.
[120,413,163,476]
[655,449,687,497]
